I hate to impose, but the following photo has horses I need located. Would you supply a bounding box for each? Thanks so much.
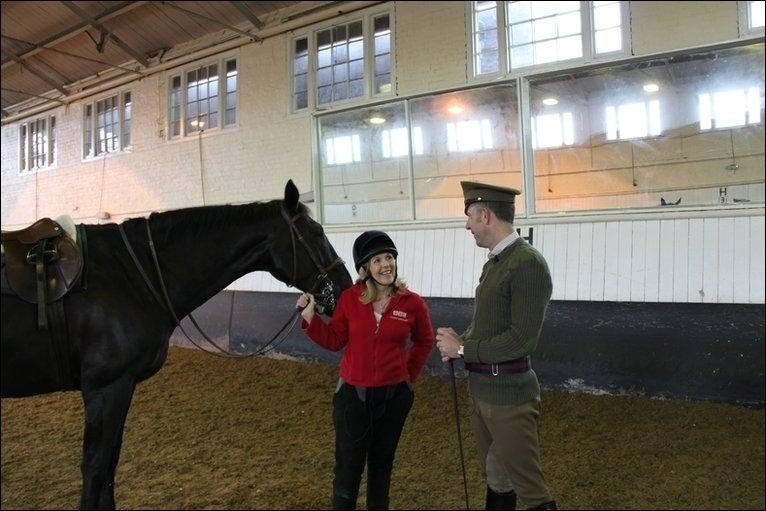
[0,179,354,511]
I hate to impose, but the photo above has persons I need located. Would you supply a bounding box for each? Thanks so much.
[295,228,436,510]
[436,181,559,510]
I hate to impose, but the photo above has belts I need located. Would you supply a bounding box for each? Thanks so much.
[464,356,532,376]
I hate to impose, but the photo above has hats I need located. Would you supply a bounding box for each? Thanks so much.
[460,180,521,214]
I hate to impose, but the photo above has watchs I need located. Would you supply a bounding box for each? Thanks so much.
[458,340,465,359]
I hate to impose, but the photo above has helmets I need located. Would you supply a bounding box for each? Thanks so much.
[352,229,399,272]
